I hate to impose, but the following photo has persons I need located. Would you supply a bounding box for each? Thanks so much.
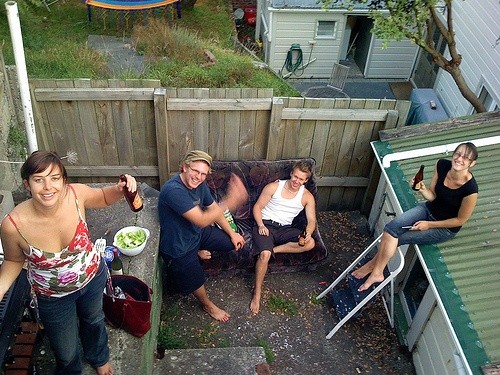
[158,150,248,322]
[250,161,316,314]
[351,142,478,291]
[0,150,137,375]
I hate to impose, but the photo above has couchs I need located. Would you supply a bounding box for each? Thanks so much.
[201,158,328,276]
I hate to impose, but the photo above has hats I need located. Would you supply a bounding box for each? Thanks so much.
[185,150,211,168]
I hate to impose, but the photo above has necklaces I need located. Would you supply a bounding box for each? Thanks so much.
[287,180,298,193]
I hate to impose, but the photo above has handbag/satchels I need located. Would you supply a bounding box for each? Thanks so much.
[103,275,153,338]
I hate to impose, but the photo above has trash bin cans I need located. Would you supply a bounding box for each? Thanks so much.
[244,6,257,25]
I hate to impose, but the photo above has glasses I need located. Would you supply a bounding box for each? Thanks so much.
[454,150,472,163]
[187,165,208,179]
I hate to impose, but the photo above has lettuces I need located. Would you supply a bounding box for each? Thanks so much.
[116,229,146,248]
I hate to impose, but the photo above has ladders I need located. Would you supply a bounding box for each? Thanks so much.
[315,233,404,339]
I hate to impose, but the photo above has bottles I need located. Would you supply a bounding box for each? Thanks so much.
[218,201,238,232]
[298,225,307,246]
[111,249,124,275]
[412,165,426,191]
[119,175,143,212]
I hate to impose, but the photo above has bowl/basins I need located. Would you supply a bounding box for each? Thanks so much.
[111,225,150,257]
